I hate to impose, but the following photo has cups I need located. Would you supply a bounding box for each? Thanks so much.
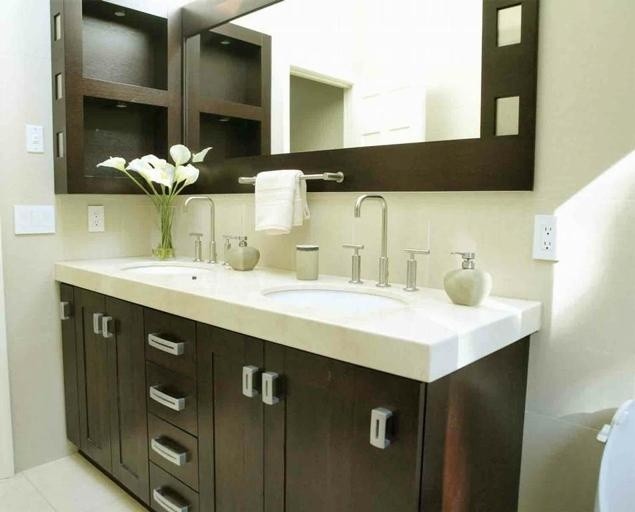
[295,245,319,280]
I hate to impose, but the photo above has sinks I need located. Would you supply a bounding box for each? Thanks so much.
[262,289,411,318]
[124,265,219,293]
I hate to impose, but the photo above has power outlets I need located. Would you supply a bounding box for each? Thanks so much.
[532,214,558,261]
[88,205,105,232]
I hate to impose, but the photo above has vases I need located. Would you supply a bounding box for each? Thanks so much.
[152,204,177,260]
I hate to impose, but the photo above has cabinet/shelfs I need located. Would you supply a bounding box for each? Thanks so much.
[285,345,426,512]
[73,288,149,506]
[50,0,181,195]
[60,282,80,449]
[148,306,200,511]
[186,22,272,159]
[214,326,287,512]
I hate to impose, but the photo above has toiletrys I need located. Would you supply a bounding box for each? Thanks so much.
[443,251,494,306]
[224,236,262,273]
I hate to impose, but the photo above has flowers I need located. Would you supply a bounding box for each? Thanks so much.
[96,143,213,247]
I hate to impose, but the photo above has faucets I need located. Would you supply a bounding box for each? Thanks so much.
[185,198,218,263]
[354,195,393,287]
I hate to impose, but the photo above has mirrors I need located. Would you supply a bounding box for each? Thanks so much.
[182,0,539,196]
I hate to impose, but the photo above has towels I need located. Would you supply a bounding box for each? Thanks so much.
[256,170,311,237]
